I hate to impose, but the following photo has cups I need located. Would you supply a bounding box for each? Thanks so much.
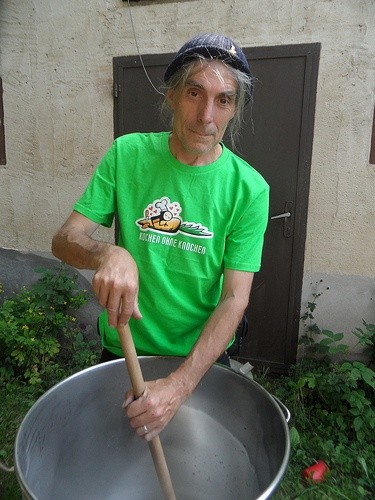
[300,460,331,487]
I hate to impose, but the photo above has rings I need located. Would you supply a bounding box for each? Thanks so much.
[142,424,148,435]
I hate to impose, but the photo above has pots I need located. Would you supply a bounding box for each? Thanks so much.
[15,356,292,500]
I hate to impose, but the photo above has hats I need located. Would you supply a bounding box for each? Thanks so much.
[163,33,256,110]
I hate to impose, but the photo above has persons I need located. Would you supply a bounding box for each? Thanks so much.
[51,33,269,441]
